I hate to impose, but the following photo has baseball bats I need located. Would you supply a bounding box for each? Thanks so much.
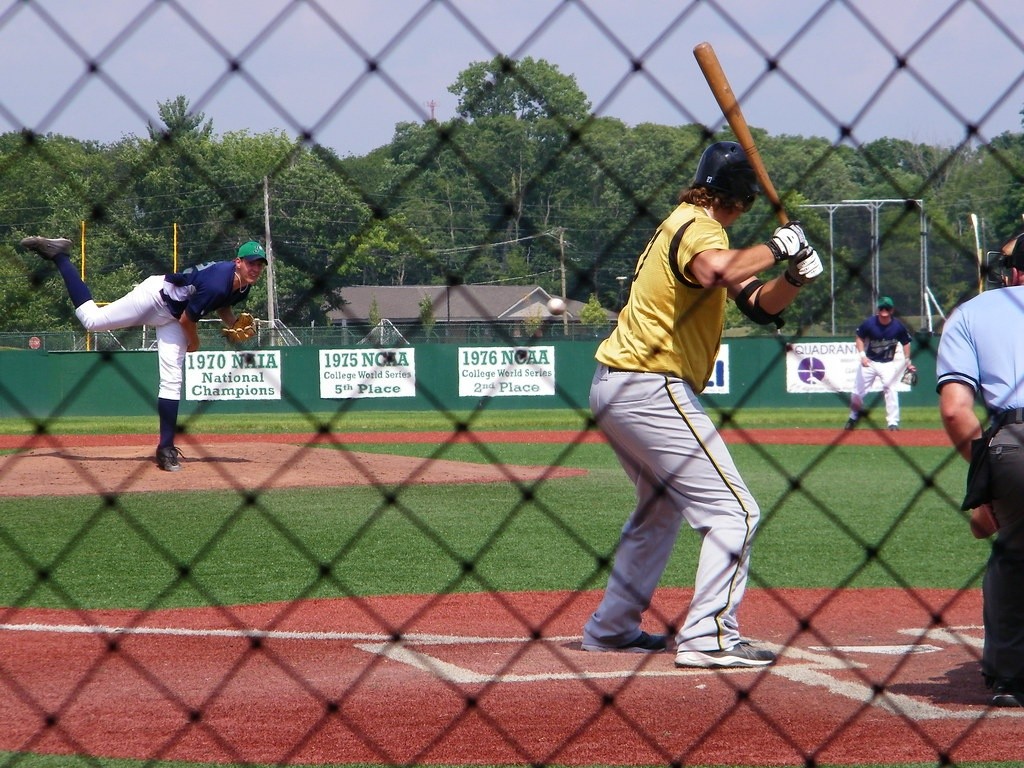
[692,41,790,228]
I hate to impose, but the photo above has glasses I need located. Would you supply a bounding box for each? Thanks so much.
[880,308,889,311]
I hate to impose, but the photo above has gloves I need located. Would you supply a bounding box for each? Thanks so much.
[784,246,823,287]
[764,221,809,263]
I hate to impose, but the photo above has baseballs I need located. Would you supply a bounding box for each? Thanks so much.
[546,298,566,315]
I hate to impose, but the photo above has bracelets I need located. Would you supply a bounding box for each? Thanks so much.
[859,351,866,358]
[905,358,911,365]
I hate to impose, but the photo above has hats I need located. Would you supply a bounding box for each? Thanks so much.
[1002,233,1024,255]
[878,297,893,308]
[238,242,268,266]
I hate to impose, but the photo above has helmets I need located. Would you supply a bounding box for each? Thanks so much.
[695,141,756,199]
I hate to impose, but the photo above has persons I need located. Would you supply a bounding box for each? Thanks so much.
[579,141,823,667]
[843,296,916,431]
[22,237,268,472]
[936,230,1024,704]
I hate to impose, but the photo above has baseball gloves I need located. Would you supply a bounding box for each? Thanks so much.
[221,313,256,344]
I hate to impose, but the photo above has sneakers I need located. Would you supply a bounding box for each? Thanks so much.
[156,444,185,471]
[21,236,73,261]
[674,642,774,667]
[581,631,665,653]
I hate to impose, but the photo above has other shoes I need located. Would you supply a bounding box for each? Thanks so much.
[845,418,856,430]
[986,679,1020,707]
[889,425,899,431]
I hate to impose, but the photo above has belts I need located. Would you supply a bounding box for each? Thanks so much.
[609,366,644,373]
[997,407,1024,425]
